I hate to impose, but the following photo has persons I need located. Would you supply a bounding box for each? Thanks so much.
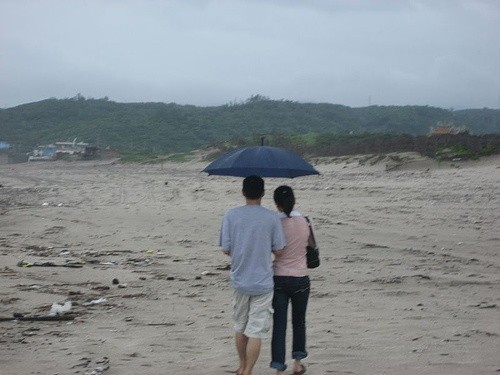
[270,185,317,375]
[218,175,286,375]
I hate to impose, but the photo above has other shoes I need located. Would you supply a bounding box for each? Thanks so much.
[294,364,306,375]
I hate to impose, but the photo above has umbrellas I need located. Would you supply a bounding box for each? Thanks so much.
[201,144,320,179]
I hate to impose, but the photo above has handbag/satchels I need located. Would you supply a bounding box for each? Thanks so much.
[305,216,319,269]
